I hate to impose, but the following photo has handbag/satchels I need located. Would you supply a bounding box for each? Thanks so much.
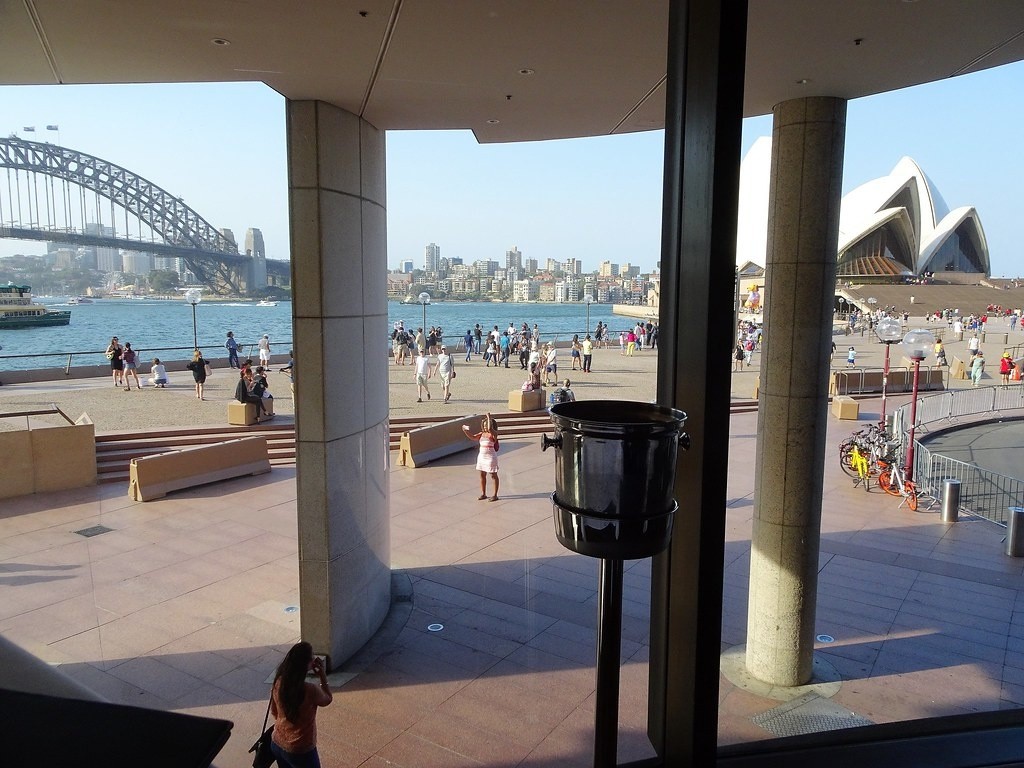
[204,364,212,376]
[107,353,114,360]
[249,724,275,768]
[133,356,141,368]
[453,372,456,378]
[938,348,945,357]
[941,358,947,365]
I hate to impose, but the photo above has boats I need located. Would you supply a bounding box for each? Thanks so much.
[256,299,278,307]
[66,296,94,305]
[0,280,71,330]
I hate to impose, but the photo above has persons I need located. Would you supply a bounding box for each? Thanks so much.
[926,307,987,338]
[391,326,454,403]
[235,360,273,425]
[1007,277,1022,289]
[562,378,575,401]
[258,333,272,372]
[571,320,659,373]
[462,413,499,502]
[968,333,985,386]
[831,341,857,368]
[906,272,934,285]
[106,336,166,391]
[850,304,910,335]
[844,281,852,288]
[272,642,333,768]
[279,350,294,401]
[734,325,762,371]
[463,322,558,386]
[224,331,241,369]
[934,339,944,368]
[191,351,210,400]
[986,303,1024,330]
[999,353,1012,390]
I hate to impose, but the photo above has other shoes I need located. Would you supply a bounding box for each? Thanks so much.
[263,411,272,416]
[417,398,422,402]
[477,495,487,500]
[489,496,498,501]
[444,399,447,403]
[427,394,430,401]
[586,370,591,373]
[447,393,451,399]
[583,370,586,372]
[255,417,261,425]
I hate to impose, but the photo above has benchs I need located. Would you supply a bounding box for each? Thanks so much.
[832,395,859,420]
[509,390,546,411]
[228,398,273,425]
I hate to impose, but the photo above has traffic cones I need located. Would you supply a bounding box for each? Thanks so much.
[1009,364,1021,381]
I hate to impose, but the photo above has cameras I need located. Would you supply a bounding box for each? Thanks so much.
[464,426,470,430]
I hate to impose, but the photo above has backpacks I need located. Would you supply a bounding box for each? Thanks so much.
[398,335,406,344]
[550,388,570,404]
[494,439,499,451]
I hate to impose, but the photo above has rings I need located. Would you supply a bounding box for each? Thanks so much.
[322,660,324,662]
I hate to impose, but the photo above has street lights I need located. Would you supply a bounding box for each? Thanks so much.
[584,294,593,338]
[846,298,854,326]
[874,317,902,432]
[418,291,431,335]
[838,298,844,320]
[900,327,936,482]
[185,288,202,351]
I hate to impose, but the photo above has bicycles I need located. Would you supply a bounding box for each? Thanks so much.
[837,419,919,511]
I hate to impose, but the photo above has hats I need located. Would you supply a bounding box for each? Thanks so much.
[547,341,555,347]
[419,349,424,352]
[1003,353,1010,357]
[441,345,447,350]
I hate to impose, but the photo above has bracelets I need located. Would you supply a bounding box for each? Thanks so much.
[320,682,329,686]
